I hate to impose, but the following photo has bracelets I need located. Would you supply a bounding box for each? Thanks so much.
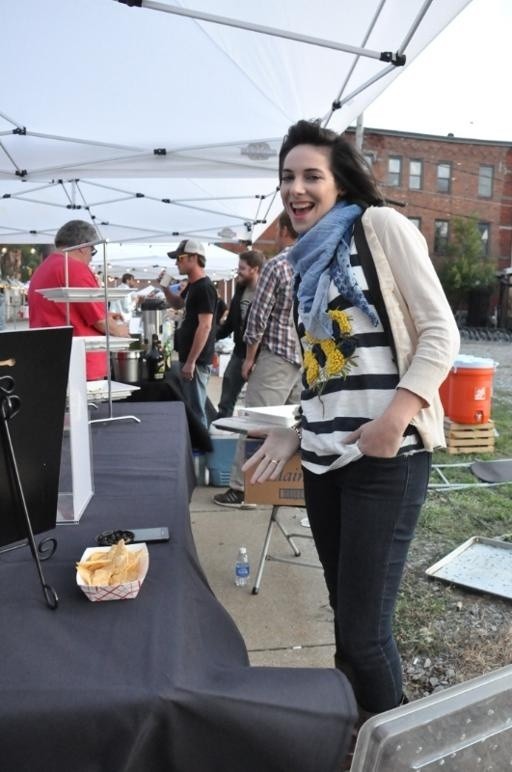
[289,424,303,441]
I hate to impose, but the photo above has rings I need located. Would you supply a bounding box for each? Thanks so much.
[270,460,279,464]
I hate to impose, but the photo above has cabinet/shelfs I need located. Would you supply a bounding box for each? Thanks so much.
[34,237,144,430]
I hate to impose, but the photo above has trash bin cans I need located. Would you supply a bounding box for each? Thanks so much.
[23,304,29,321]
[440,354,494,424]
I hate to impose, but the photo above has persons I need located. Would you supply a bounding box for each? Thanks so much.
[156,236,217,456]
[210,206,307,510]
[233,118,464,772]
[180,277,189,293]
[210,288,229,357]
[214,249,268,420]
[108,271,141,323]
[26,218,134,383]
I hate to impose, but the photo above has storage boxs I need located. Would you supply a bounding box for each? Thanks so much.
[241,437,306,508]
[207,436,241,487]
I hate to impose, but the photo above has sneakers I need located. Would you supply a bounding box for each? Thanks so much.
[211,488,259,510]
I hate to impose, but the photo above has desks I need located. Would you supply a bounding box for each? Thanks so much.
[1,402,186,771]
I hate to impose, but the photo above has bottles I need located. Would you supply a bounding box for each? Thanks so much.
[234,548,250,586]
[143,333,170,381]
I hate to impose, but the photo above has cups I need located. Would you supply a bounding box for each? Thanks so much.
[160,274,173,288]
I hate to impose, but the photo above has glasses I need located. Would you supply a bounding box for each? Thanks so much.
[89,244,98,256]
[176,256,188,260]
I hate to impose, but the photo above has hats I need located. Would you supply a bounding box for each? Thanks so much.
[168,238,207,260]
